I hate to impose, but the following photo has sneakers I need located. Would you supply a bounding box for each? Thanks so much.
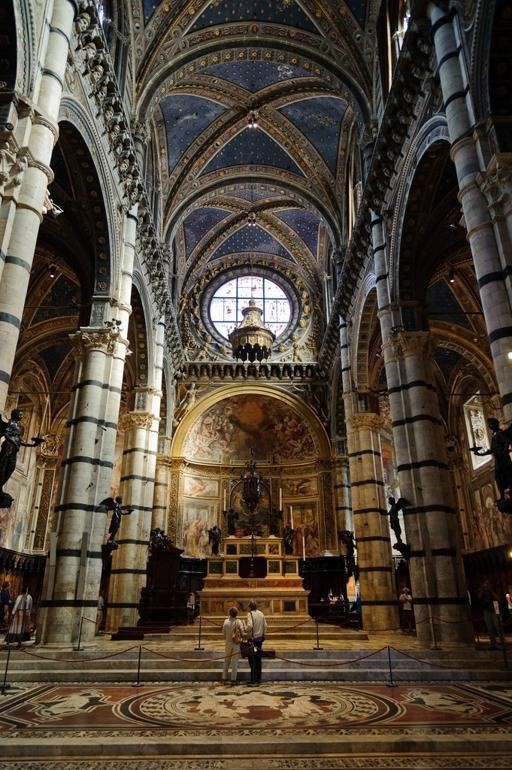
[220,679,240,685]
[246,679,263,684]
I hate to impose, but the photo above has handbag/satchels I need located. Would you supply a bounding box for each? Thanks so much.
[240,639,258,659]
[232,618,247,644]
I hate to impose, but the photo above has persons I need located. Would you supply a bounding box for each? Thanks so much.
[0,406,38,498]
[208,525,222,556]
[94,589,105,636]
[0,580,12,628]
[477,581,508,645]
[107,494,134,544]
[291,379,329,422]
[504,584,512,621]
[339,530,357,566]
[3,585,32,651]
[398,587,414,634]
[473,416,512,502]
[226,508,239,533]
[221,607,248,683]
[176,375,214,421]
[379,496,403,545]
[282,523,295,555]
[243,599,268,683]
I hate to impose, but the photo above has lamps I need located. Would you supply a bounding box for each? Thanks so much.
[227,181,276,364]
